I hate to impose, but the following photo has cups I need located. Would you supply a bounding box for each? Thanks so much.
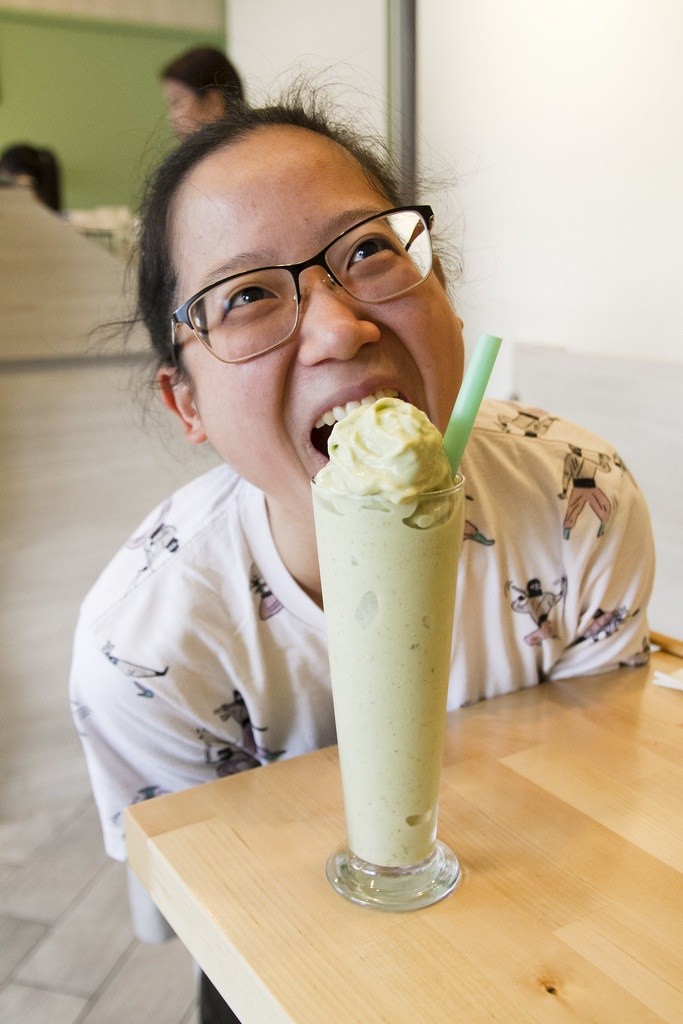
[311,473,467,913]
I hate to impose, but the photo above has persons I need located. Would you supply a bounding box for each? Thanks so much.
[0,141,63,228]
[62,103,657,1023]
[161,45,246,147]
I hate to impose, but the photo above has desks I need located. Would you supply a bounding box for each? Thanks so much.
[1,176,142,356]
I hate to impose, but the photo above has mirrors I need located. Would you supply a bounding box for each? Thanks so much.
[1,2,418,368]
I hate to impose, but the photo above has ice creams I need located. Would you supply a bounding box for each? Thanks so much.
[311,398,466,870]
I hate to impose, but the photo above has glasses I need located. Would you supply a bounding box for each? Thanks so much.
[170,204,435,363]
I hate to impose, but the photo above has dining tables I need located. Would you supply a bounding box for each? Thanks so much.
[121,651,683,1024]
